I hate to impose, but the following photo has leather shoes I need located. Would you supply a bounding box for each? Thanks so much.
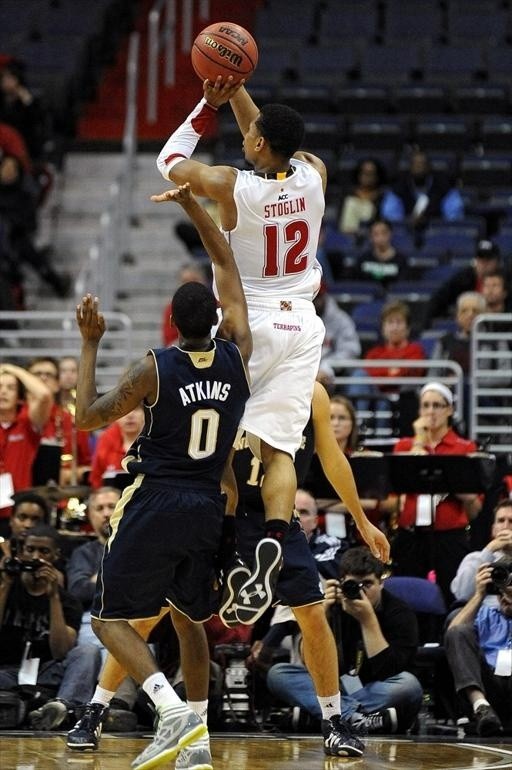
[234,537,284,626]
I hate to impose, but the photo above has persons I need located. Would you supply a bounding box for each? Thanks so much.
[0,493,66,592]
[132,372,391,758]
[443,555,512,738]
[1,525,100,732]
[309,395,373,499]
[88,400,147,490]
[76,181,256,770]
[245,480,350,689]
[267,547,423,736]
[442,499,512,633]
[0,363,55,540]
[55,357,94,484]
[154,74,327,632]
[392,382,484,606]
[22,356,74,497]
[27,486,165,733]
[1,52,74,299]
[161,258,214,347]
[141,609,253,731]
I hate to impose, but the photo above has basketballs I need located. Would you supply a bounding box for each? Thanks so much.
[189,20,260,88]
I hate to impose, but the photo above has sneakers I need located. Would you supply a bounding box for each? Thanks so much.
[267,705,300,733]
[175,740,214,770]
[28,697,69,731]
[354,707,398,736]
[106,700,137,733]
[219,557,252,630]
[321,715,365,758]
[130,702,208,770]
[474,704,504,736]
[66,701,112,749]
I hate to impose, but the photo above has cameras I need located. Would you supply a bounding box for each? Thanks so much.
[483,560,511,596]
[3,558,41,576]
[338,579,367,603]
